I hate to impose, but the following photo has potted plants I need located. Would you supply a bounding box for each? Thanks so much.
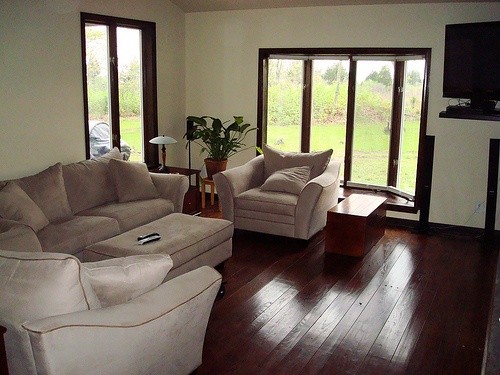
[182,116,263,178]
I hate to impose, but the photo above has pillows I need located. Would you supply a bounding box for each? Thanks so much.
[261,166,312,194]
[108,158,160,202]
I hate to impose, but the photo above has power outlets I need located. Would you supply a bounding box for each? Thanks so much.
[475,200,483,213]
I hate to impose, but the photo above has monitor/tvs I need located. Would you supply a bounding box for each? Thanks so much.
[441,21,500,112]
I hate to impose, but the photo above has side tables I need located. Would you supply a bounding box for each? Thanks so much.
[202,177,221,210]
[151,166,202,216]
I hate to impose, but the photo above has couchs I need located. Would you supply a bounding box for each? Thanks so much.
[212,144,340,239]
[0,146,222,375]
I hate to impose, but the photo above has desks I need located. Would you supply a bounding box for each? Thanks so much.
[326,193,388,257]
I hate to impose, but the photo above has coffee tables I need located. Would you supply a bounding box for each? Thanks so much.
[83,213,234,282]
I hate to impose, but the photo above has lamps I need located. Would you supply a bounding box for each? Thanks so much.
[149,135,177,173]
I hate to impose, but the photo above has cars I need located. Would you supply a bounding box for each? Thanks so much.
[89,120,131,161]
